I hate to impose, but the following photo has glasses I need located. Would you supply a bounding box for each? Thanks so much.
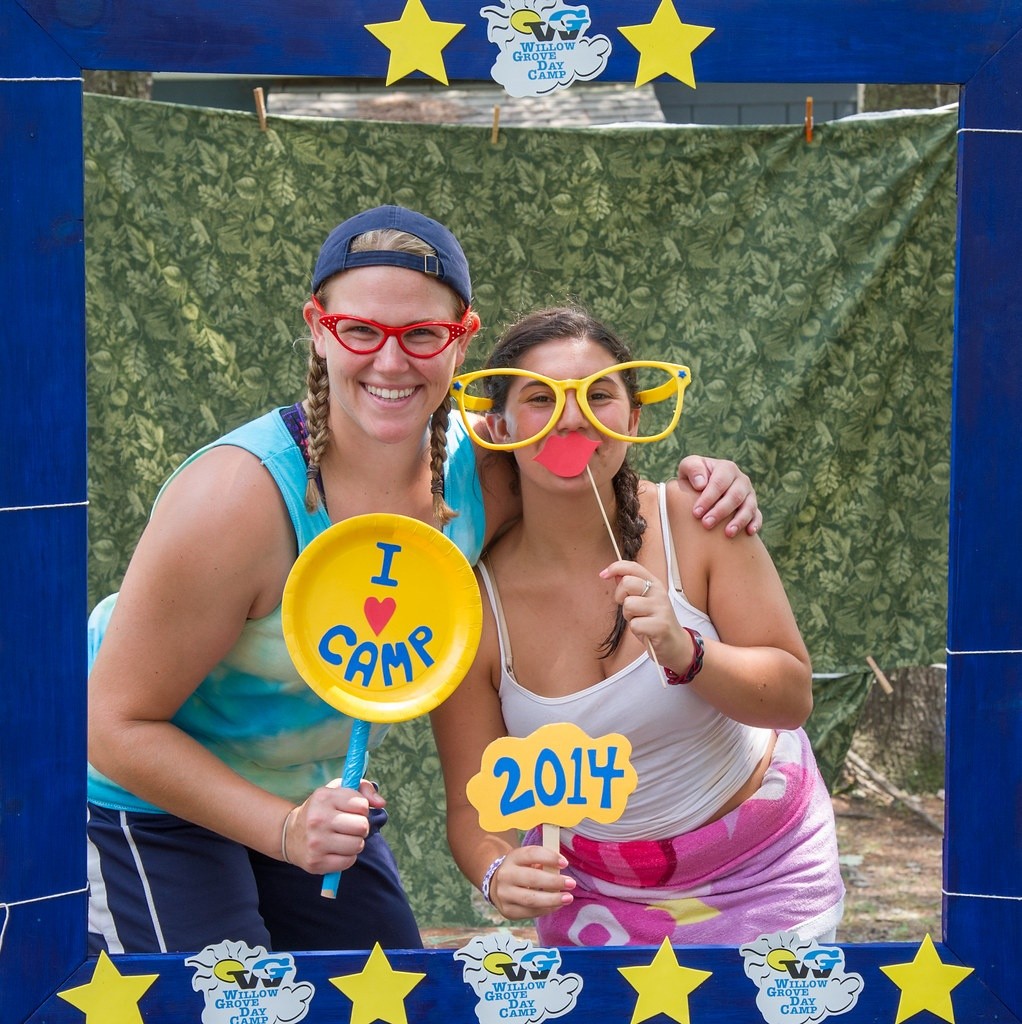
[309,294,472,358]
[451,358,691,450]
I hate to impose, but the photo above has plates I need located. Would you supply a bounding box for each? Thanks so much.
[278,511,486,734]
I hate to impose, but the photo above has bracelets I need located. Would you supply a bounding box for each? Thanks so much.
[664,626,706,687]
[281,804,301,865]
[482,852,508,910]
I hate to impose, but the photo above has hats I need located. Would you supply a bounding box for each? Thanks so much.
[312,205,472,308]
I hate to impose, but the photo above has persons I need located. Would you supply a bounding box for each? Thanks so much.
[429,303,844,949]
[86,202,765,952]
